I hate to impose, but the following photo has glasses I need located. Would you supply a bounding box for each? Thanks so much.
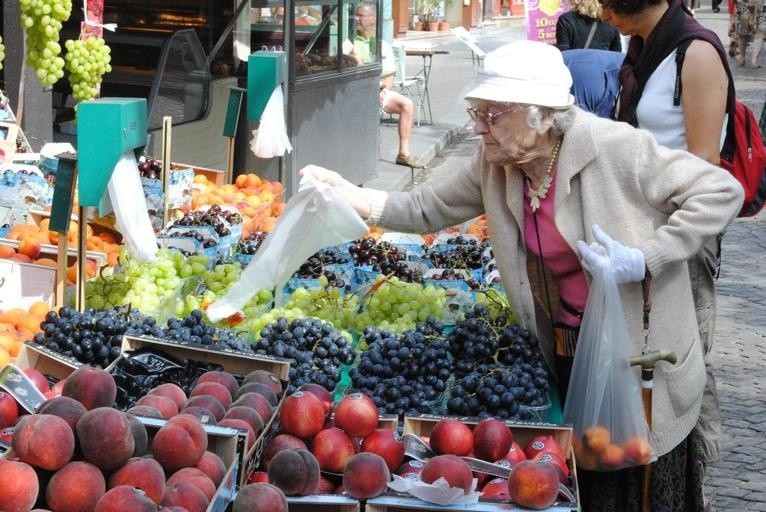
[466,105,522,123]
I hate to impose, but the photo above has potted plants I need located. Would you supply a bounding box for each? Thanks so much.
[414,0,452,31]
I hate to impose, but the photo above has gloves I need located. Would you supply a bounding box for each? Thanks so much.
[578,225,646,286]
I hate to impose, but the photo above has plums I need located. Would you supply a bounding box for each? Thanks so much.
[0,301,50,368]
[168,173,284,241]
[0,198,121,283]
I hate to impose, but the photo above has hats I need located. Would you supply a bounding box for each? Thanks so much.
[465,42,575,111]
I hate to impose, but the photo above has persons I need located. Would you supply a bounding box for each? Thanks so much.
[687,0,762,69]
[556,0,622,53]
[597,0,734,469]
[355,0,425,169]
[560,48,627,121]
[297,38,749,512]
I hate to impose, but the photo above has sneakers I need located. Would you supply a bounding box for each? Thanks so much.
[396,154,427,171]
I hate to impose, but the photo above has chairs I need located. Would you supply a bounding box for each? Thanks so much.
[391,46,429,127]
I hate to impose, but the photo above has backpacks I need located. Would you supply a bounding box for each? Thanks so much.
[674,35,766,219]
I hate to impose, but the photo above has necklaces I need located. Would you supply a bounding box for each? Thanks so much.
[526,139,561,213]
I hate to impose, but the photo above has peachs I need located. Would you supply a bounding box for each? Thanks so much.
[0,365,652,512]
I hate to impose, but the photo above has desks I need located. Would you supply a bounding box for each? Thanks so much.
[404,50,450,124]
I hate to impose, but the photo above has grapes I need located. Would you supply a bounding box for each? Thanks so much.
[35,205,549,421]
[137,157,163,179]
[64,37,112,113]
[19,0,73,87]
[0,36,6,70]
